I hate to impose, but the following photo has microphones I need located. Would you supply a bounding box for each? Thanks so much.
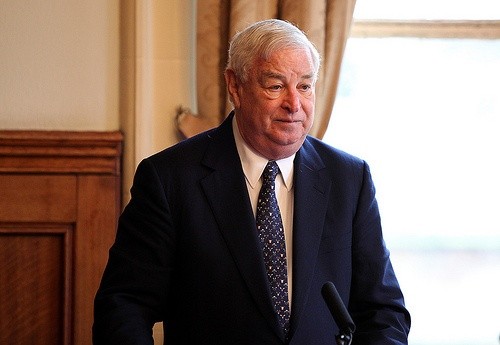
[321,282,355,333]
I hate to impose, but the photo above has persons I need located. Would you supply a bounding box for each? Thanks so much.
[92,19,411,345]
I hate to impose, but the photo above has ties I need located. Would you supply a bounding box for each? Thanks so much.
[256,161,290,345]
